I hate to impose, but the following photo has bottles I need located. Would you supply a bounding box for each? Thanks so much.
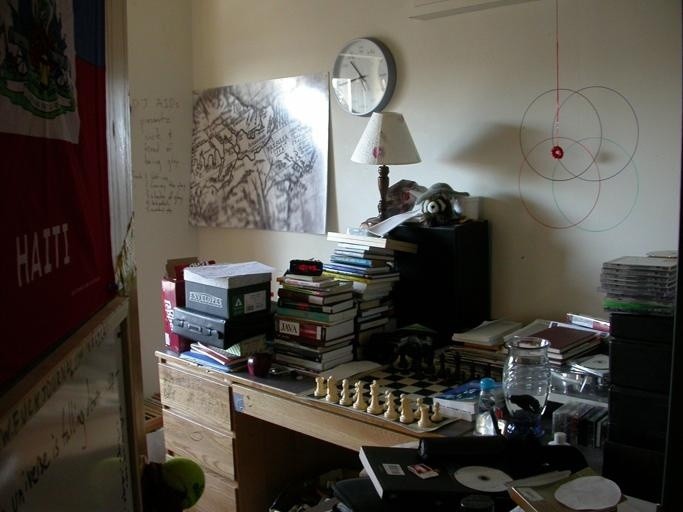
[501,336,552,438]
[473,377,497,437]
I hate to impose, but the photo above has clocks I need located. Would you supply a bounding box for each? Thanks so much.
[331,37,397,117]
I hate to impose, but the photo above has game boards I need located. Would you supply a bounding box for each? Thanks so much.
[308,344,500,434]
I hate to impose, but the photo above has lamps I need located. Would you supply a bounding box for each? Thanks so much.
[350,112,422,226]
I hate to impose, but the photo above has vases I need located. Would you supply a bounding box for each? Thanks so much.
[502,336,552,423]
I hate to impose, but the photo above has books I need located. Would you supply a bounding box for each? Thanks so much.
[360,442,470,502]
[174,230,421,381]
[453,310,605,369]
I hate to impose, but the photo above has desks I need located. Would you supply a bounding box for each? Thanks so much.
[154,350,475,512]
